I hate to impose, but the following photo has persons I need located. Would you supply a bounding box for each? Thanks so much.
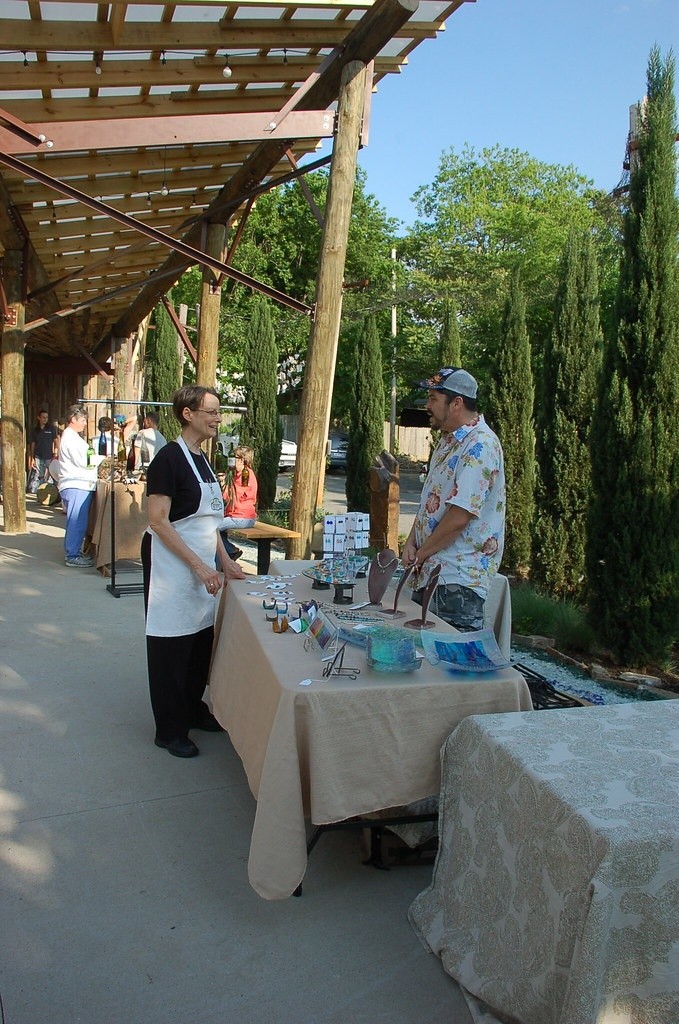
[401,366,507,632]
[140,386,246,758]
[214,446,257,572]
[27,404,167,568]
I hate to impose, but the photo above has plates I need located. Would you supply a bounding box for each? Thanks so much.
[335,622,423,650]
[421,627,520,672]
[365,635,422,673]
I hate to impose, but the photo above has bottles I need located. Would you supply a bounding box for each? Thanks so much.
[87,439,94,467]
[117,431,126,463]
[98,428,107,456]
[242,460,249,487]
[214,442,225,474]
[141,433,150,466]
[127,439,135,470]
[227,442,237,472]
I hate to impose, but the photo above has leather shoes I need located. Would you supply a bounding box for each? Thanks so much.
[154,734,199,758]
[191,712,224,732]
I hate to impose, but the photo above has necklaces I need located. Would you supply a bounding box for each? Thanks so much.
[376,552,447,617]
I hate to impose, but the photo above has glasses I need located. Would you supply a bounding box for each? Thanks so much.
[189,408,223,417]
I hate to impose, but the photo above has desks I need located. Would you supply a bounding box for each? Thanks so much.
[407,698,678,1024]
[220,522,302,575]
[202,561,536,903]
[86,465,149,577]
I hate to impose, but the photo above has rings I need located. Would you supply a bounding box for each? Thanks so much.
[211,584,214,586]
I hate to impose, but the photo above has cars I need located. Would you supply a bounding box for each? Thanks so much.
[326,432,350,475]
[219,424,297,471]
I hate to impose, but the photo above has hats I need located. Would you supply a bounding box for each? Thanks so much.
[413,366,479,401]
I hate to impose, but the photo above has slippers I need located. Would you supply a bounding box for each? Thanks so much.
[220,550,243,573]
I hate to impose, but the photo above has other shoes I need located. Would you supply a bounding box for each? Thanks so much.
[65,550,94,568]
